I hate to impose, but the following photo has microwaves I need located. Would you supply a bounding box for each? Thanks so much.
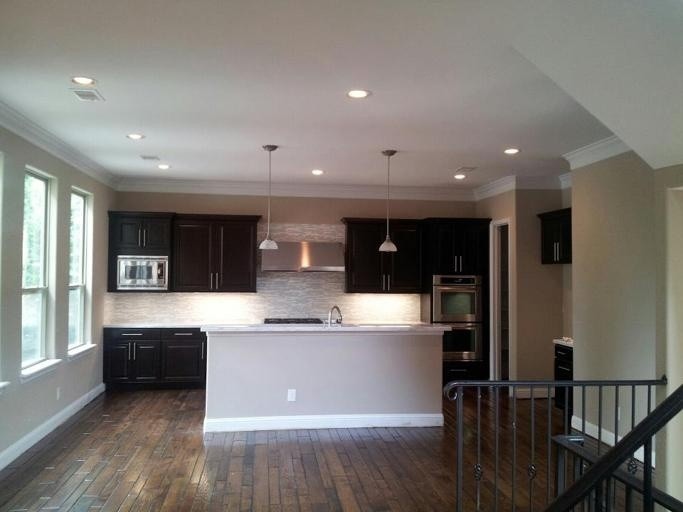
[117,254,169,291]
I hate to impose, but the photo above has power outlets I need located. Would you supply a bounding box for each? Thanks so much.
[57,387,63,401]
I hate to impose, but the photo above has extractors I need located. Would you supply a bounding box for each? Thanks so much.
[260,241,346,272]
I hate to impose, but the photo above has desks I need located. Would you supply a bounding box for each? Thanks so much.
[200,329,444,432]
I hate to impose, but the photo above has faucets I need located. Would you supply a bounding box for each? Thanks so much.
[328,305,343,325]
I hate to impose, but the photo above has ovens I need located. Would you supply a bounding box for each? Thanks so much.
[431,274,484,323]
[434,324,484,363]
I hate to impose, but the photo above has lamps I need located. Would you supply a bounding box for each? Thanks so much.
[254,145,406,255]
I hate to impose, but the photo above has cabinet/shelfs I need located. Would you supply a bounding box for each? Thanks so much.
[102,329,160,391]
[345,218,424,293]
[553,345,574,412]
[160,329,207,389]
[173,212,257,294]
[538,205,573,266]
[424,217,492,293]
[444,358,487,380]
[107,211,173,258]
